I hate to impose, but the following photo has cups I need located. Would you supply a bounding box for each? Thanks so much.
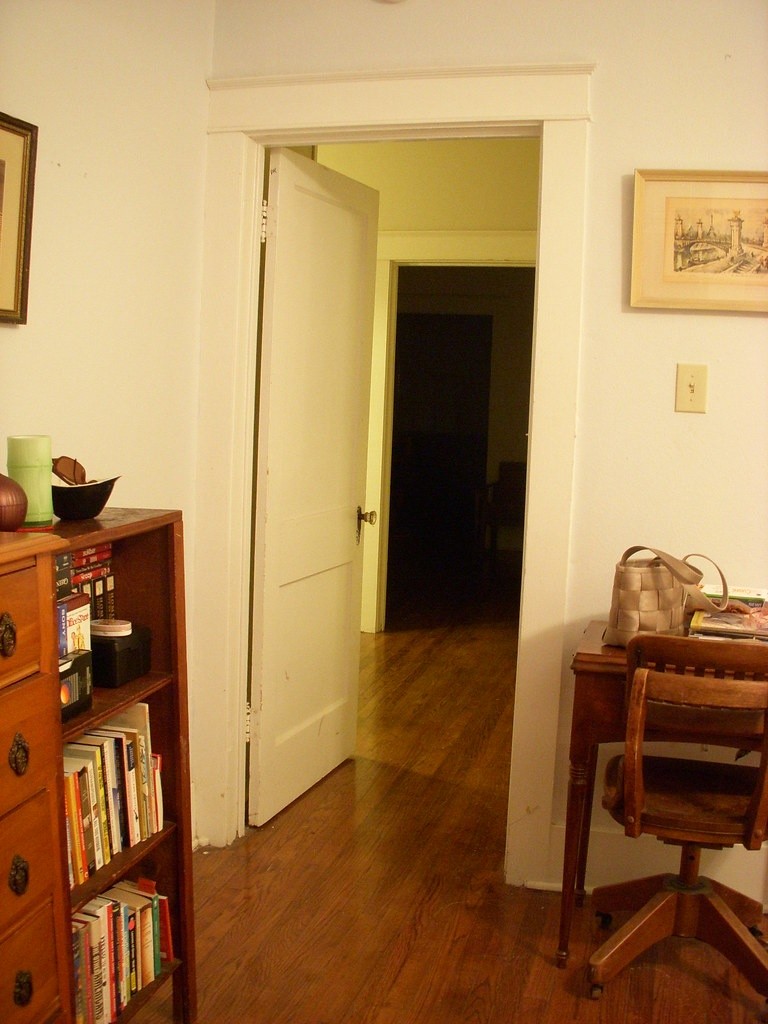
[6,435,54,528]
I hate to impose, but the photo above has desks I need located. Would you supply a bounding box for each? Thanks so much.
[555,620,754,969]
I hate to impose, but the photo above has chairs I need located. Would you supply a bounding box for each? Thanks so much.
[588,634,768,1000]
[473,460,526,571]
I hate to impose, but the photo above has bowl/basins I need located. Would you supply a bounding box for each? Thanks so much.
[52,476,120,519]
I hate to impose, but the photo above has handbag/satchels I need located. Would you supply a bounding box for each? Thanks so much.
[601,546,729,644]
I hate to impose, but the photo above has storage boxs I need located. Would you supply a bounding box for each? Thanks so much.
[89,624,151,688]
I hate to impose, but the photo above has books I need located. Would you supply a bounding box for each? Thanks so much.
[53,543,116,660]
[63,703,164,891]
[690,583,768,641]
[72,880,174,1024]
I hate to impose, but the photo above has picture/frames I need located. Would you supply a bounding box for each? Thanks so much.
[629,168,768,312]
[0,110,39,324]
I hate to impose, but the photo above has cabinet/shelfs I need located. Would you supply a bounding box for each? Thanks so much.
[0,507,198,1024]
